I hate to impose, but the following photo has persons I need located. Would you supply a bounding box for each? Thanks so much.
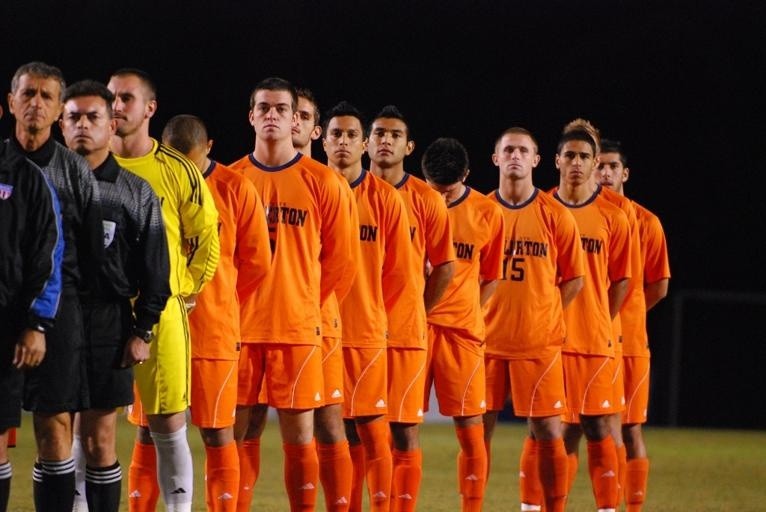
[350,106,455,512]
[519,130,632,512]
[560,136,671,512]
[225,80,350,512]
[321,101,411,512]
[126,114,271,512]
[0,61,221,512]
[546,118,639,512]
[456,127,586,512]
[389,136,504,512]
[244,88,361,512]
